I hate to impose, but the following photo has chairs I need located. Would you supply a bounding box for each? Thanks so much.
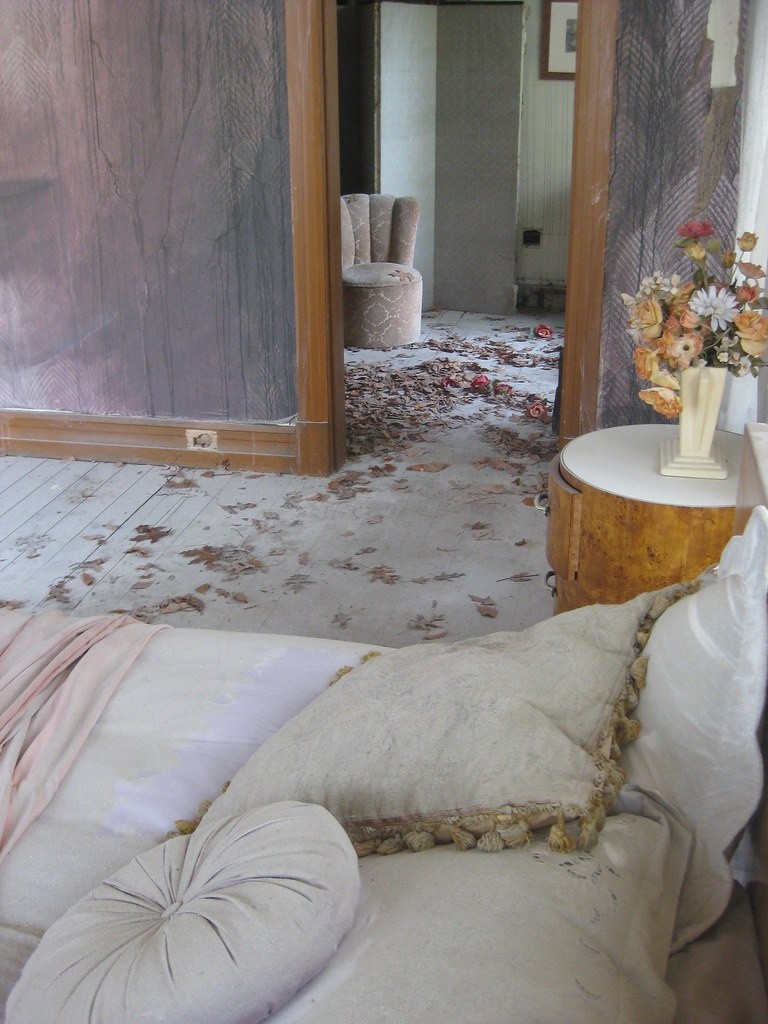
[338,195,422,348]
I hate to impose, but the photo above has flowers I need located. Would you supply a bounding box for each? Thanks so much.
[622,220,768,416]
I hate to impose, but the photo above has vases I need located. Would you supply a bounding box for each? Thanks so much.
[660,363,730,479]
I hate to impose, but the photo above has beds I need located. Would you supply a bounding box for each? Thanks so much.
[0,604,768,1023]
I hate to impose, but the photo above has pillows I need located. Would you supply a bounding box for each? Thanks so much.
[266,782,703,1023]
[596,506,768,954]
[168,575,700,863]
[5,792,357,1024]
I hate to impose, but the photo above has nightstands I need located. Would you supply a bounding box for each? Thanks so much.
[534,428,745,617]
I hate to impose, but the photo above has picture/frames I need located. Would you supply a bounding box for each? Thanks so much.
[541,0,580,79]
